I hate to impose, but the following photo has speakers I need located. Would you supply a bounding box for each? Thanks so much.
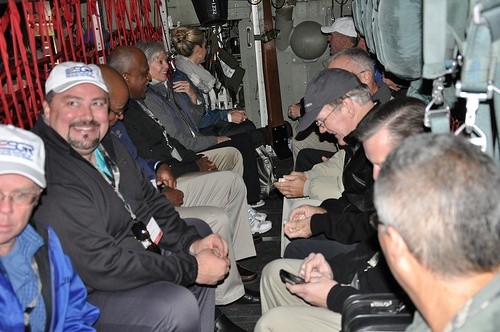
[272,121,293,160]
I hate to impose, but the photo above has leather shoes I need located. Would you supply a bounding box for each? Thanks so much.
[236,263,257,282]
[219,287,261,307]
[214,313,247,332]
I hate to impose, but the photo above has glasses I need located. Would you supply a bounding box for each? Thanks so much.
[316,106,338,129]
[0,190,41,206]
[369,212,386,228]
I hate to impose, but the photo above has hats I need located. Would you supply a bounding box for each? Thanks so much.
[295,67,362,133]
[45,61,110,94]
[321,17,357,38]
[0,124,47,189]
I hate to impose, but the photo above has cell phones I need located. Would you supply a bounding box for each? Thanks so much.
[280,269,305,284]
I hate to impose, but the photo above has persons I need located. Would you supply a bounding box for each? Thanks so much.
[254,96,431,332]
[374,133,500,332]
[0,124,100,332]
[99,65,261,332]
[110,24,278,281]
[26,62,230,332]
[273,16,399,259]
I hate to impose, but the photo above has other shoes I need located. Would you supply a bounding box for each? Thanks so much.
[252,233,262,245]
[247,212,272,234]
[248,206,271,222]
[248,200,265,208]
